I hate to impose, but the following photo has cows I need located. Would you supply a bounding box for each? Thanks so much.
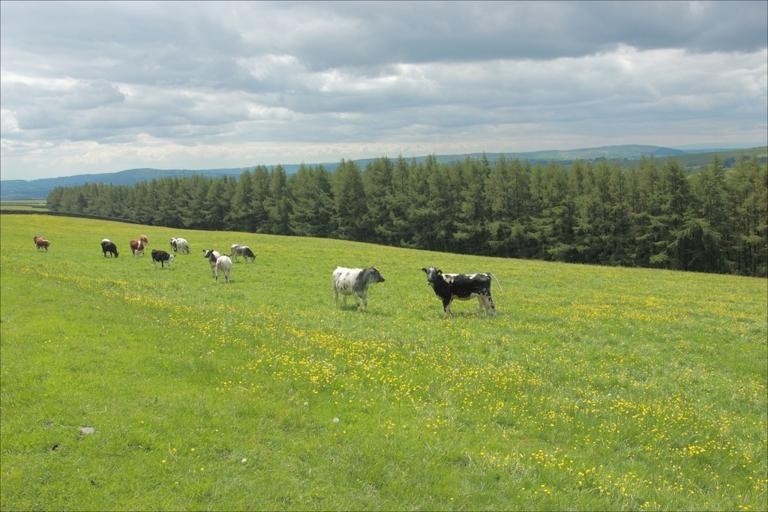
[33,236,50,251]
[151,248,175,268]
[420,267,503,317]
[100,238,120,258]
[214,253,232,284]
[332,265,386,313]
[170,238,190,255]
[202,248,220,278]
[230,244,258,262]
[129,234,148,256]
